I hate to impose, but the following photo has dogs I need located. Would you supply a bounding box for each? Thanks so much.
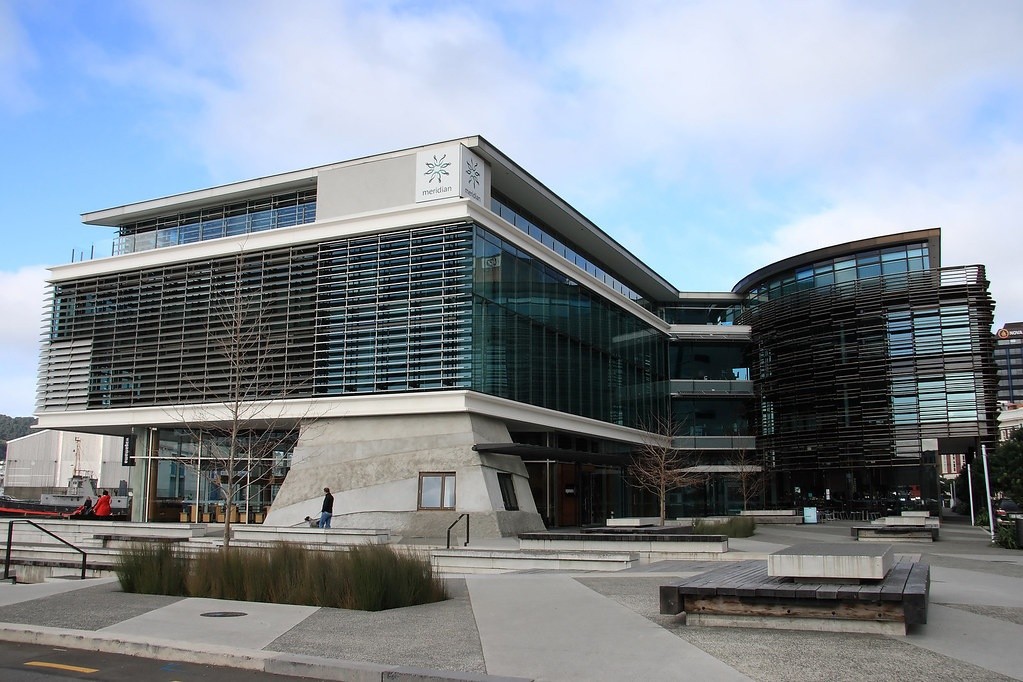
[305,516,325,528]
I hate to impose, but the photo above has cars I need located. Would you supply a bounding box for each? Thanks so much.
[992,497,1019,527]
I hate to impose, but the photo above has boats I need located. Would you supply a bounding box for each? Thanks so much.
[0,437,129,519]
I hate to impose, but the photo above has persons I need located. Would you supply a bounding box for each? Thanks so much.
[318,487,334,528]
[80,490,114,521]
